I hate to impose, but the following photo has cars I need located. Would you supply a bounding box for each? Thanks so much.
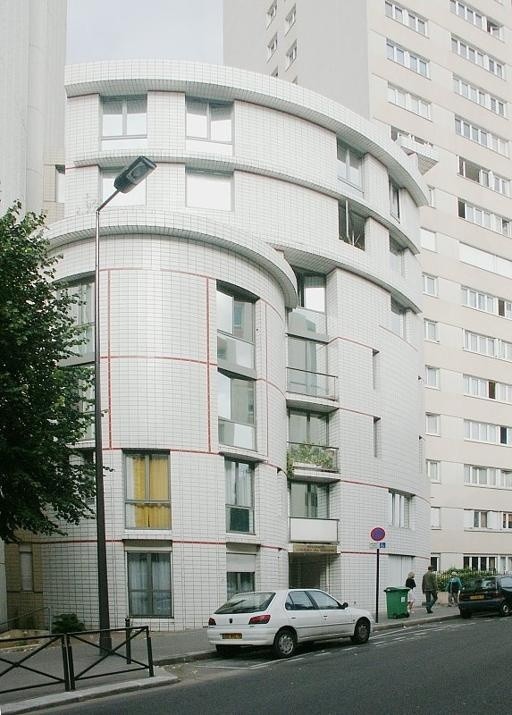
[207,589,373,658]
[458,575,512,619]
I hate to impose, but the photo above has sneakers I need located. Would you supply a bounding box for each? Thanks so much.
[425,607,432,614]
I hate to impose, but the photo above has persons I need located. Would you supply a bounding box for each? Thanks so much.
[404,571,417,615]
[421,564,441,613]
[445,570,462,606]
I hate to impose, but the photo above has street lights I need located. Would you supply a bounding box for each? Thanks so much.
[95,156,156,655]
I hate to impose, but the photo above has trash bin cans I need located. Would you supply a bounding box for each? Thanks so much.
[384,586,411,619]
